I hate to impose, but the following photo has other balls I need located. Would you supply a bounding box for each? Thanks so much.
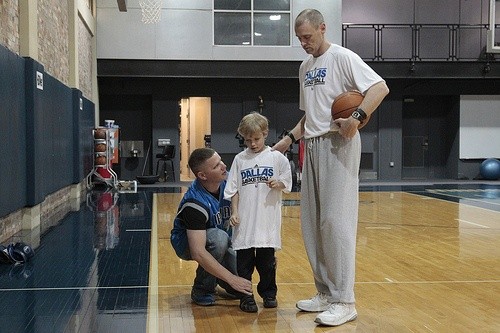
[479,158,500,181]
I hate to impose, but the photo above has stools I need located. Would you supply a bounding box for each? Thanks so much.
[156,145,176,182]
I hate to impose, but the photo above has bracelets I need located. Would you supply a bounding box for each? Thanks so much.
[356,108,367,120]
[288,132,295,143]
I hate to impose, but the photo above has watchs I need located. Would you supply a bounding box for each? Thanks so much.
[352,110,363,124]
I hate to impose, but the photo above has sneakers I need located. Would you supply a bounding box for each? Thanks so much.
[314,301,358,326]
[296,293,333,312]
[191,290,217,306]
[263,296,277,308]
[240,297,258,312]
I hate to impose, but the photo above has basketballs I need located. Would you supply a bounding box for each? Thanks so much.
[94,143,106,152]
[94,130,106,139]
[95,155,106,165]
[330,90,371,129]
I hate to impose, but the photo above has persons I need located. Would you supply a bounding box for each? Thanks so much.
[270,8,389,327]
[223,113,293,312]
[170,148,254,305]
